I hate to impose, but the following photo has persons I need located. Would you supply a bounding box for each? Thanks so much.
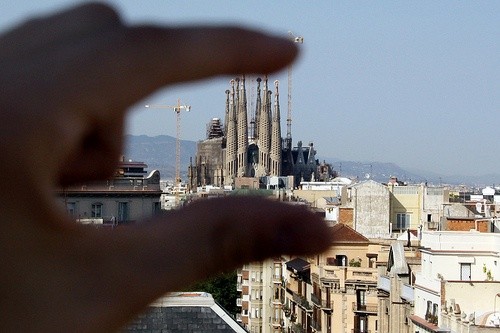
[0,3,331,333]
